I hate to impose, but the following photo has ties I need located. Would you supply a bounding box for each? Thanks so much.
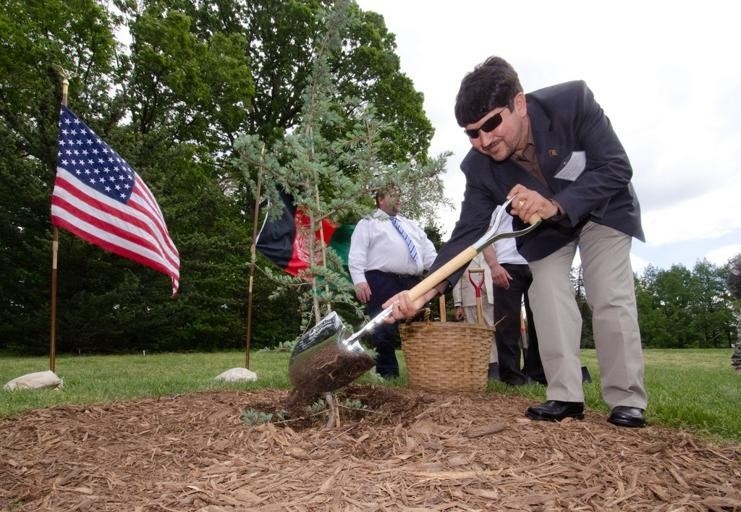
[389,216,419,261]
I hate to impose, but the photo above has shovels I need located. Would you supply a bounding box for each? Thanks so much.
[289,196,542,401]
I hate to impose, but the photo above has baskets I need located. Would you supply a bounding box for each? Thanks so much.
[398,322,497,396]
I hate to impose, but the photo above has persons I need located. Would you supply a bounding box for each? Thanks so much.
[481,204,547,387]
[452,253,502,380]
[380,56,649,428]
[354,184,438,381]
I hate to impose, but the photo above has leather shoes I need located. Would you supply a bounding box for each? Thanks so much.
[525,400,584,420]
[609,406,644,426]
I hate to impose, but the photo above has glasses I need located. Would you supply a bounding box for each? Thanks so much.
[463,105,508,139]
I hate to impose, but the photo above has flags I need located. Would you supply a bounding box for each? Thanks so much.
[253,183,357,295]
[49,103,182,300]
